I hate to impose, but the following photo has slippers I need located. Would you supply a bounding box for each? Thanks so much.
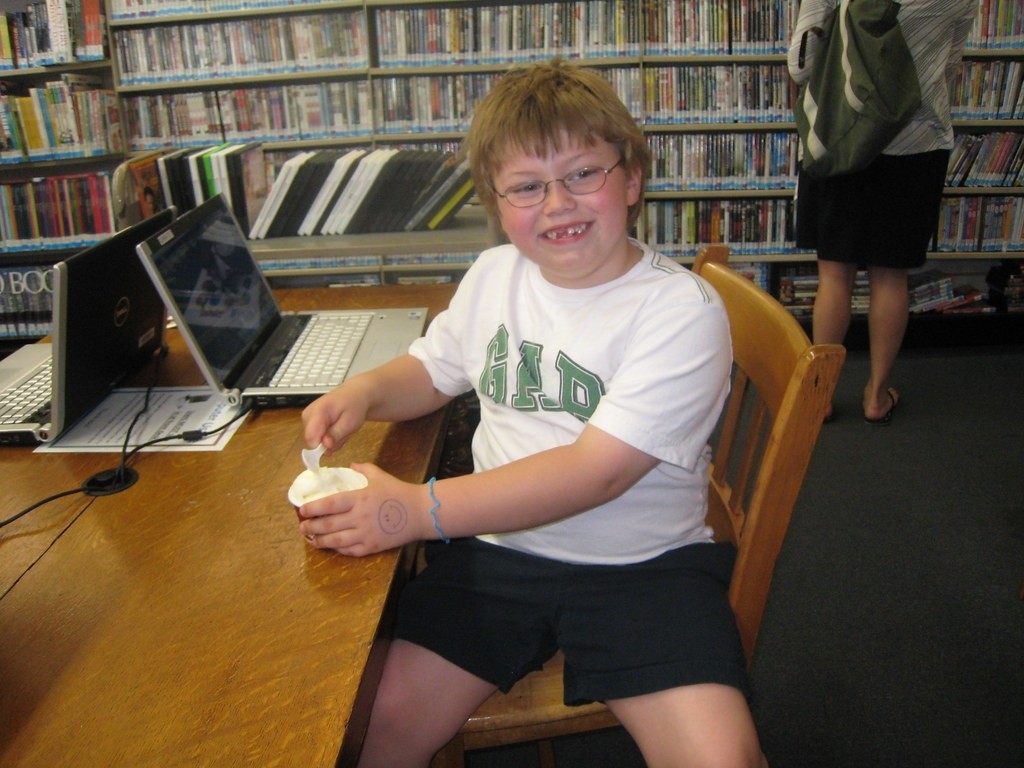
[860,384,901,425]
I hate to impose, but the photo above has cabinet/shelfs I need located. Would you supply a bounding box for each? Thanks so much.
[0,1,1024,340]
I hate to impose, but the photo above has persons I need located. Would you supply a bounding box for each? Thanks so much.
[293,59,767,768]
[781,0,980,424]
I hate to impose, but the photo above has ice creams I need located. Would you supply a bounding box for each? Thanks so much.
[288,466,368,507]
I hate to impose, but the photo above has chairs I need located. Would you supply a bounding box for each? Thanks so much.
[429,240,849,768]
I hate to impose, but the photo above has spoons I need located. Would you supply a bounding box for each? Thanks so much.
[300,443,327,476]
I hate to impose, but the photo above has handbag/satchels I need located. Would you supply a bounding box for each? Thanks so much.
[787,0,923,181]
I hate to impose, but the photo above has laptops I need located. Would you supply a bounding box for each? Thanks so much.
[1,206,178,447]
[136,193,429,408]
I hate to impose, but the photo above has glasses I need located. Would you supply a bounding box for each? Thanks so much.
[488,152,627,209]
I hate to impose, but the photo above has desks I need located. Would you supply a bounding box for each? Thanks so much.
[2,283,455,767]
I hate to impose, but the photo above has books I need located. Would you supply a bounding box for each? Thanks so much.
[1,0,1024,322]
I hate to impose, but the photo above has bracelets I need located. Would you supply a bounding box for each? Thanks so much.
[427,476,450,545]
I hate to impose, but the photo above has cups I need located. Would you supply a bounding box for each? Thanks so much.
[288,466,368,542]
